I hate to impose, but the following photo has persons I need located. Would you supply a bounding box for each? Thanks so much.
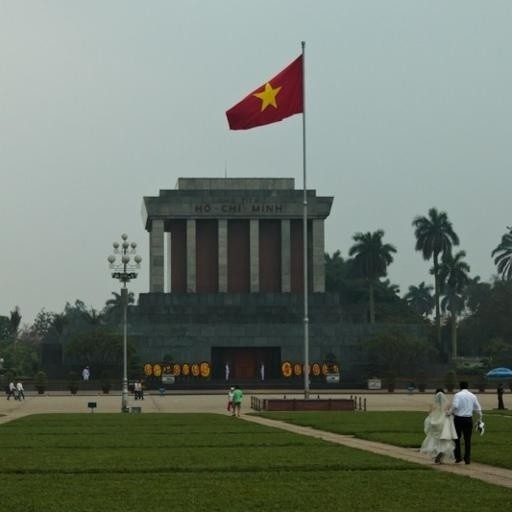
[81,365,90,384]
[139,377,146,400]
[134,379,142,399]
[496,382,505,409]
[231,384,243,417]
[227,386,234,411]
[445,380,485,465]
[17,378,26,400]
[6,378,16,401]
[418,387,458,466]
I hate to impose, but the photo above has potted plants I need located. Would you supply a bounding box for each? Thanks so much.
[384,369,487,392]
[35,371,111,394]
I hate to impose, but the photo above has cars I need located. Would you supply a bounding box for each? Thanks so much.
[486,367,511,378]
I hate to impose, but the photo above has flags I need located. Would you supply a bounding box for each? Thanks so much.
[225,53,304,130]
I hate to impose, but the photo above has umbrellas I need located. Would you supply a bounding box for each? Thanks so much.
[485,367,512,380]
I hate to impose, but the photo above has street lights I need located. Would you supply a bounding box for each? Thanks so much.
[107,231,144,413]
